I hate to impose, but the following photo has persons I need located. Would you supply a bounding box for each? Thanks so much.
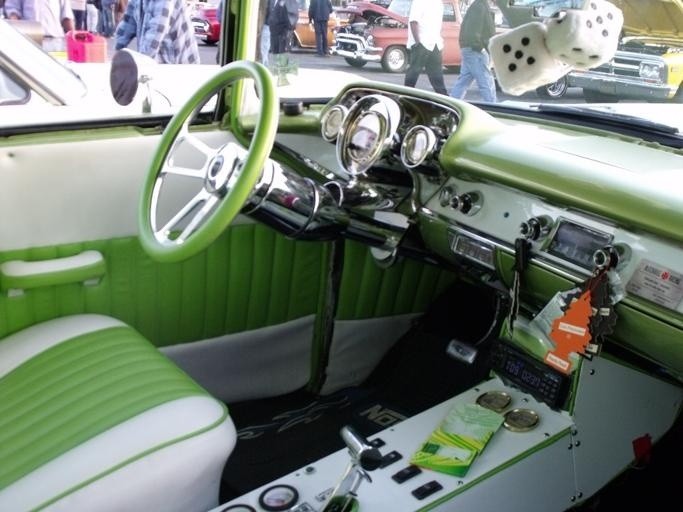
[402,0,448,96]
[0,0,334,67]
[449,1,499,105]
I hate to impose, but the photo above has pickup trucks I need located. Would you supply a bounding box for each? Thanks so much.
[326,0,462,74]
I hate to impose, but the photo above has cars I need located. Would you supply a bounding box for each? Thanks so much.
[536,0,683,103]
[284,1,346,53]
[184,1,221,45]
[0,14,368,128]
[495,0,584,99]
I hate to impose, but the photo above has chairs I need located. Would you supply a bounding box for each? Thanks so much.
[0,314,237,512]
